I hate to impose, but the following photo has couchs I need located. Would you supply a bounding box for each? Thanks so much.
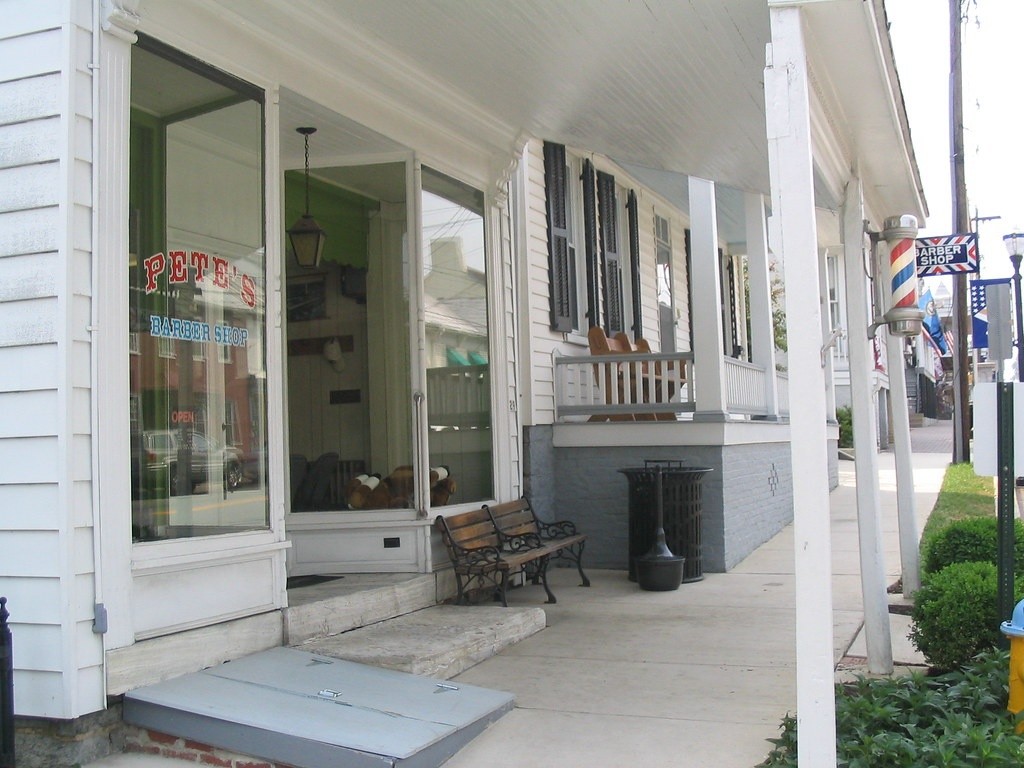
[369,466,456,509]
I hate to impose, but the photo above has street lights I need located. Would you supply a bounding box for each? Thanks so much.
[1002,223,1023,384]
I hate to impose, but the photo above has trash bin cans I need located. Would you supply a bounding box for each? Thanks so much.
[616,464,713,585]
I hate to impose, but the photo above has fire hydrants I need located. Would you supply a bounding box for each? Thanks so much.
[1000,598,1024,736]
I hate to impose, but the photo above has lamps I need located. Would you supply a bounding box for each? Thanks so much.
[286,126,329,270]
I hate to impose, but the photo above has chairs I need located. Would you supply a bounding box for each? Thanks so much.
[290,454,309,504]
[295,453,339,511]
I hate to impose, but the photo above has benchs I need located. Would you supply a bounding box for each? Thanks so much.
[436,496,591,607]
[588,327,686,422]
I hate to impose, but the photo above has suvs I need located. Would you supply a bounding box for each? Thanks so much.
[132,427,247,496]
